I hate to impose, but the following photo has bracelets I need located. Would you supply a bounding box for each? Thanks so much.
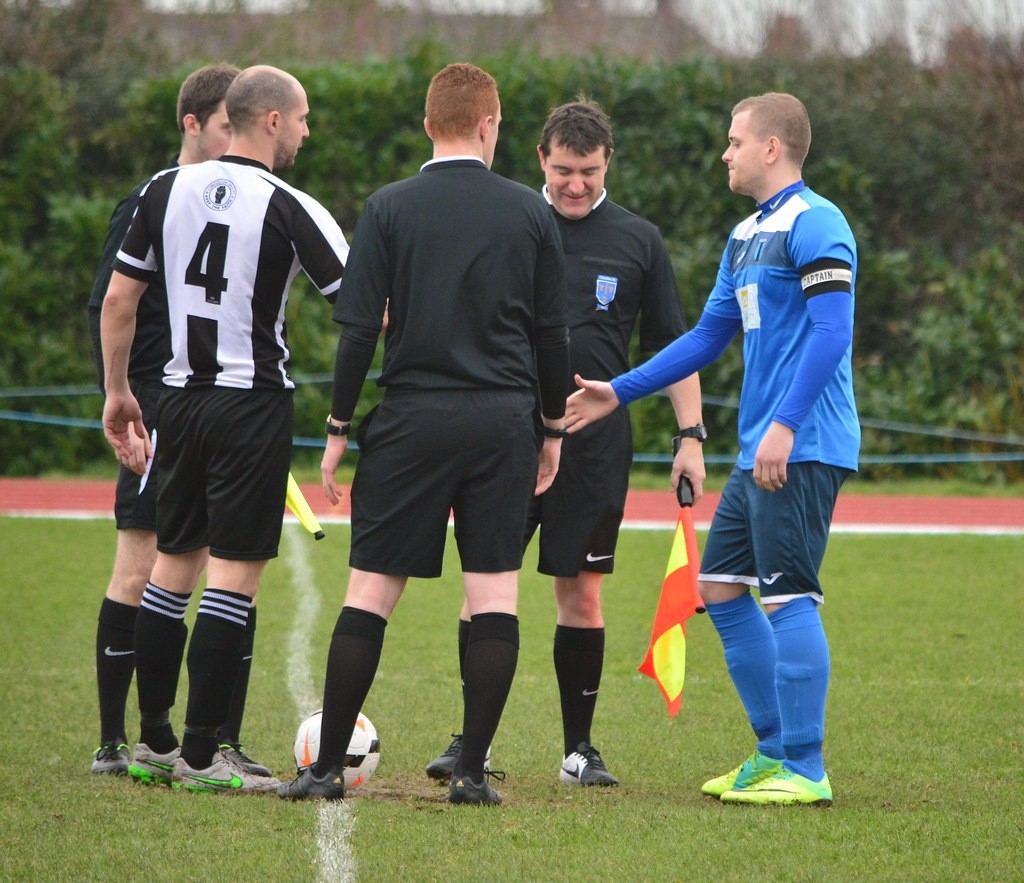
[547,426,569,438]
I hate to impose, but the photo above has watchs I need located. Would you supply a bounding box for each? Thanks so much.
[679,424,707,442]
[326,415,352,435]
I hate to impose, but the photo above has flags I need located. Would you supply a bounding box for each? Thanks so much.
[285,474,321,534]
[637,507,704,718]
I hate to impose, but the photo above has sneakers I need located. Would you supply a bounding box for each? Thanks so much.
[426,734,493,777]
[450,767,507,806]
[172,753,282,796]
[89,737,131,776]
[276,761,346,799]
[720,768,834,808]
[219,742,273,776]
[127,742,183,785]
[559,746,619,788]
[701,749,784,798]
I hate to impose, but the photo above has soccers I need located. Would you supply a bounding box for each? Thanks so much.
[293,708,381,789]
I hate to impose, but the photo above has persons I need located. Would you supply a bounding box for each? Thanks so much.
[566,94,861,809]
[424,102,707,786]
[94,63,243,773]
[100,65,390,794]
[276,64,569,803]
